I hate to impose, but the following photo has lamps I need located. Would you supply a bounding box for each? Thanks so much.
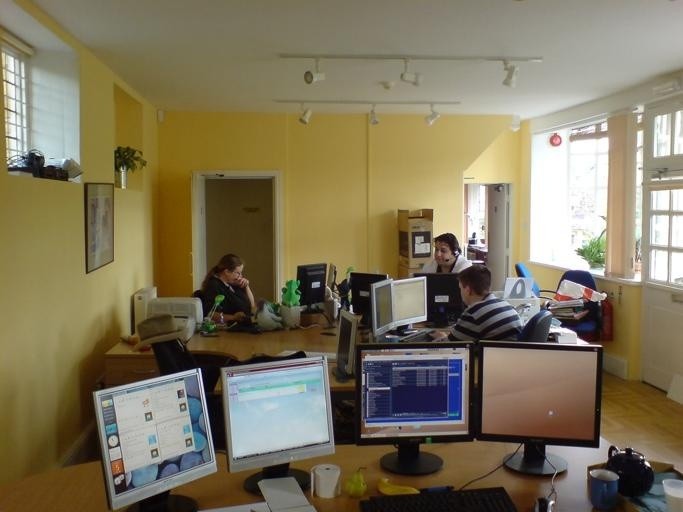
[297,104,312,125]
[201,294,225,332]
[303,59,326,85]
[501,64,518,88]
[397,62,426,87]
[346,264,354,304]
[369,105,379,127]
[423,104,441,125]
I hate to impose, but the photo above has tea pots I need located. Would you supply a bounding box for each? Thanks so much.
[603,445,654,498]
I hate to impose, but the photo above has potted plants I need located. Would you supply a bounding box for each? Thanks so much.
[575,235,607,269]
[114,144,148,190]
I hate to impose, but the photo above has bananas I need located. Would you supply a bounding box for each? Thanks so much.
[378,477,421,496]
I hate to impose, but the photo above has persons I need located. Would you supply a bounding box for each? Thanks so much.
[428,265,522,341]
[422,233,472,274]
[201,253,255,322]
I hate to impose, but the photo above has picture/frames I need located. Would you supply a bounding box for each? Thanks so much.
[84,182,115,275]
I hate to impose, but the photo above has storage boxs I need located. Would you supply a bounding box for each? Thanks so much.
[398,208,434,269]
[398,264,434,279]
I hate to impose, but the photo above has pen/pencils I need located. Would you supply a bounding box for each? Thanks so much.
[418,484,454,493]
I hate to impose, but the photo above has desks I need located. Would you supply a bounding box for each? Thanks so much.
[102,312,592,397]
[0,439,683,512]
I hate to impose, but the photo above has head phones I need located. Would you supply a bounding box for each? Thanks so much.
[445,249,460,262]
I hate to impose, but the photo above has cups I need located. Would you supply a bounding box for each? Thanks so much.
[662,478,682,511]
[588,468,619,512]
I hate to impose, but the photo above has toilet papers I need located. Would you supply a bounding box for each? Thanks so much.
[309,464,341,499]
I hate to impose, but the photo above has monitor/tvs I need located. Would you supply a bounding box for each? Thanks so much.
[332,310,358,383]
[350,273,388,315]
[371,278,396,337]
[297,263,327,314]
[474,340,603,478]
[93,367,219,511]
[327,262,338,293]
[355,341,475,476]
[413,273,464,328]
[390,276,427,335]
[221,355,336,496]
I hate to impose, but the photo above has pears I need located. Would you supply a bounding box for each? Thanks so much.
[345,467,368,497]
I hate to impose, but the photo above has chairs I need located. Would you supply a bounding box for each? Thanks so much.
[515,310,553,343]
[514,261,558,301]
[554,267,601,342]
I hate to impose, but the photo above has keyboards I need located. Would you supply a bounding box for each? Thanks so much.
[359,486,516,511]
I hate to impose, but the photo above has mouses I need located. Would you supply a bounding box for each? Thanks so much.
[535,496,551,512]
